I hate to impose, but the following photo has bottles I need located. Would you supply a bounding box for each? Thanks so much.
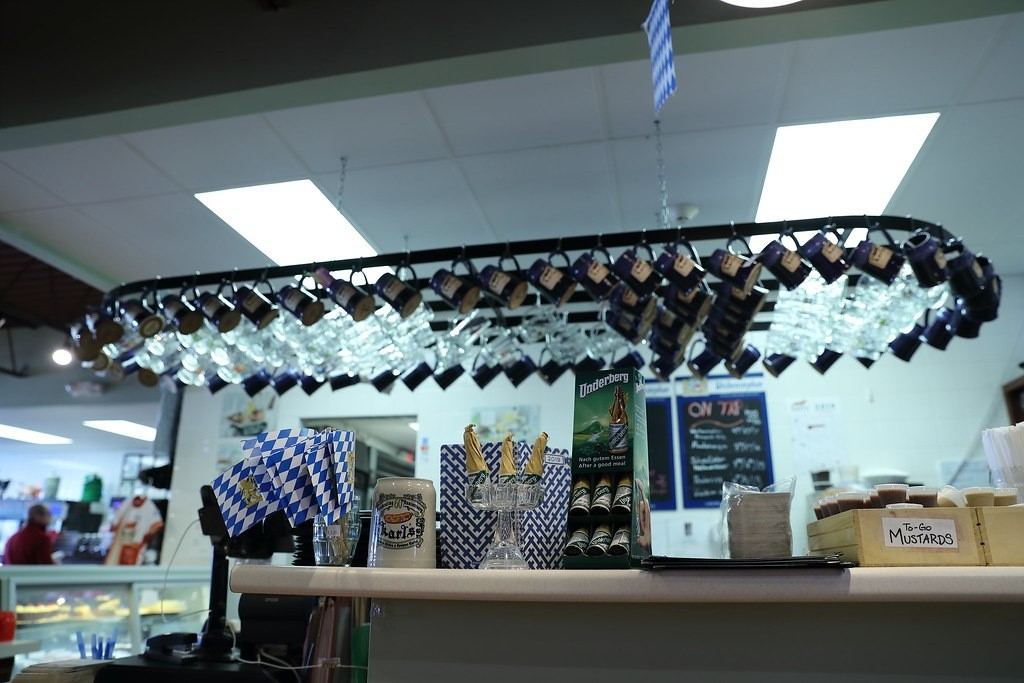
[571,477,590,515]
[564,523,589,556]
[522,431,549,487]
[589,475,612,514]
[586,524,610,557]
[498,433,517,486]
[612,475,634,514]
[608,521,632,556]
[464,423,490,501]
[606,386,630,453]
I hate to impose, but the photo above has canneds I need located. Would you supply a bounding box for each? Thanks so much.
[368,478,436,569]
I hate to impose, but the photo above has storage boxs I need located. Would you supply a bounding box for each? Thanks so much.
[439,444,571,570]
[807,507,1000,568]
[976,507,1023,566]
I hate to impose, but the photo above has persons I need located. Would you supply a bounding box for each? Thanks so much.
[100,481,163,566]
[1,505,61,565]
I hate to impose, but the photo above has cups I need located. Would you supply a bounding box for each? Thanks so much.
[312,494,363,568]
[813,484,1018,520]
[65,229,1002,394]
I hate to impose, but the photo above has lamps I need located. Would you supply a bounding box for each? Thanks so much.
[51,338,74,367]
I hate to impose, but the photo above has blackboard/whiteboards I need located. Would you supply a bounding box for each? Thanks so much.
[677,392,774,508]
[646,397,677,511]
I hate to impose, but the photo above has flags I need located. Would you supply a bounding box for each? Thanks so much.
[211,427,357,539]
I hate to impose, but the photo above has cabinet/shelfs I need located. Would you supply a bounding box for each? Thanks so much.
[0,565,213,683]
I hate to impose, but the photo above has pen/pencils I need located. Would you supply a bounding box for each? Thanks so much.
[76,631,86,658]
[91,627,119,659]
